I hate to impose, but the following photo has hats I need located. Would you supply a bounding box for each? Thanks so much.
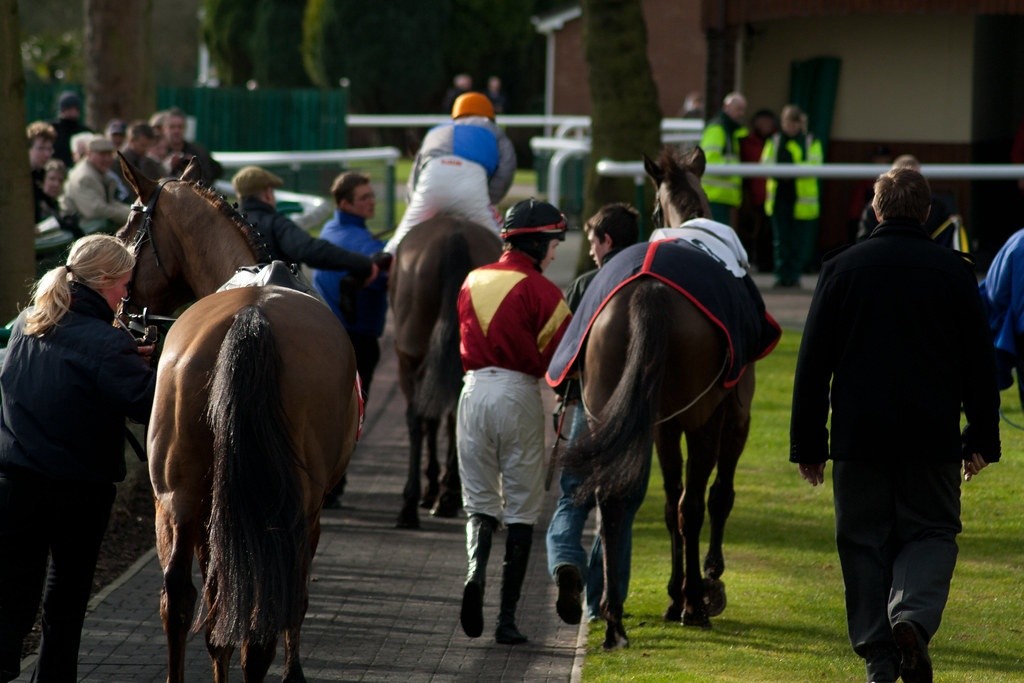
[233,167,283,195]
[110,121,126,136]
[89,139,115,152]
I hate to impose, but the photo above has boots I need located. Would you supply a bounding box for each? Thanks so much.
[496,524,533,644]
[460,514,492,638]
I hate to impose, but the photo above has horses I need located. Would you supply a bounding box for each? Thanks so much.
[116,148,363,683]
[552,146,756,632]
[387,215,504,529]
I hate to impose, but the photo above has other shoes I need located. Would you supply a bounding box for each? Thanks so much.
[588,613,601,623]
[556,566,583,625]
[892,620,933,683]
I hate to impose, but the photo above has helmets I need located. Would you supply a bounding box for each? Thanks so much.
[500,198,568,241]
[451,92,495,121]
[60,93,83,111]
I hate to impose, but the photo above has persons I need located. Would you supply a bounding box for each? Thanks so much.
[0,68,1024,683]
